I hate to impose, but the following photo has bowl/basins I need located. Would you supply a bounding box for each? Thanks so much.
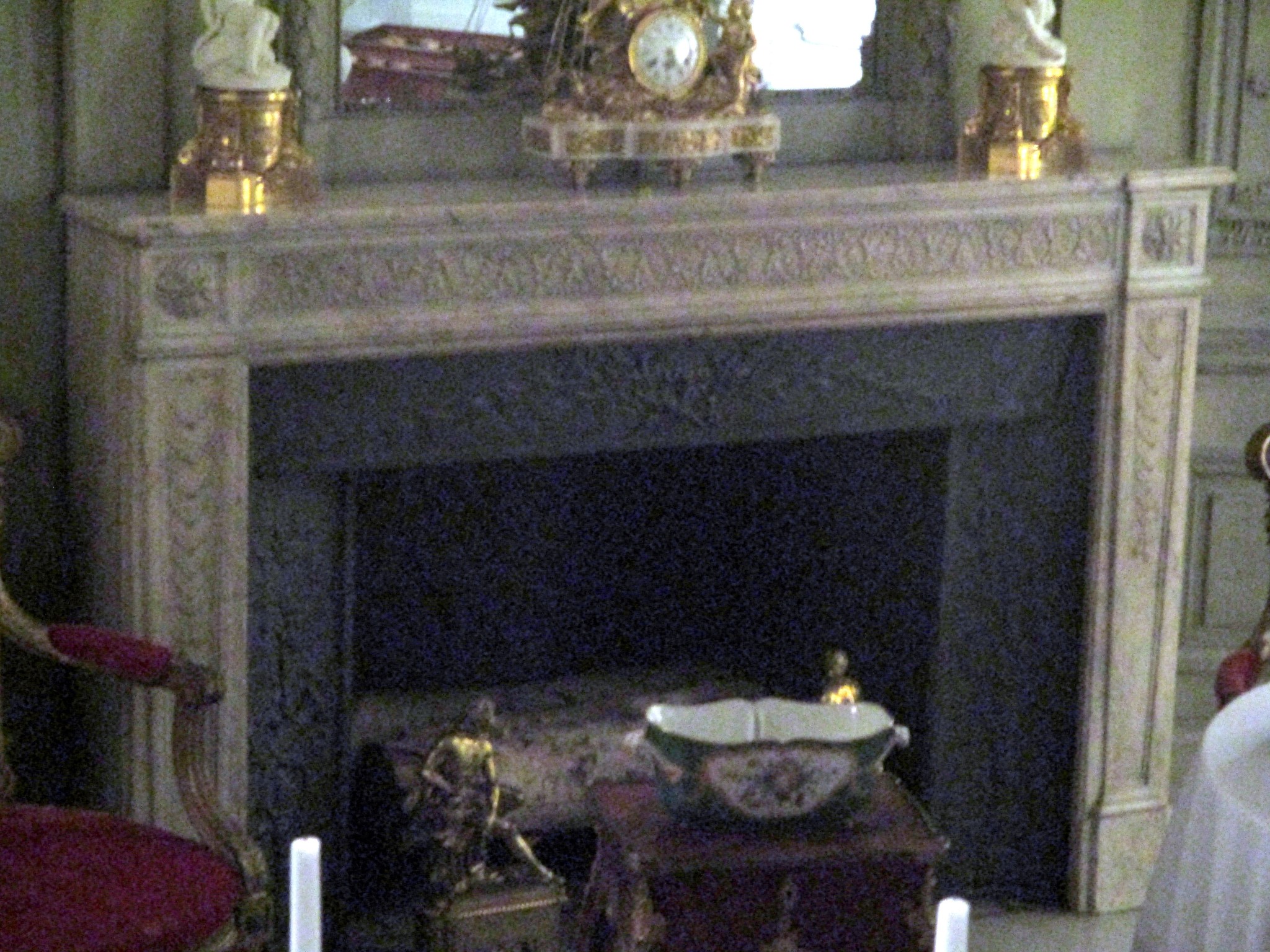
[643,700,909,837]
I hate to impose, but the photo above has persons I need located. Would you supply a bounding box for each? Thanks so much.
[415,696,565,917]
[819,649,863,707]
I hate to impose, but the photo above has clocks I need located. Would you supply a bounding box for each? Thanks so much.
[518,0,781,192]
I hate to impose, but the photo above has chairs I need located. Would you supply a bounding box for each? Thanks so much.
[0,585,273,951]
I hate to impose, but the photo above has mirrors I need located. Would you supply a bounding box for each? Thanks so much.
[333,0,880,116]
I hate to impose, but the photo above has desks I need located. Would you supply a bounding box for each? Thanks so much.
[577,768,951,952]
[1129,682,1270,951]
